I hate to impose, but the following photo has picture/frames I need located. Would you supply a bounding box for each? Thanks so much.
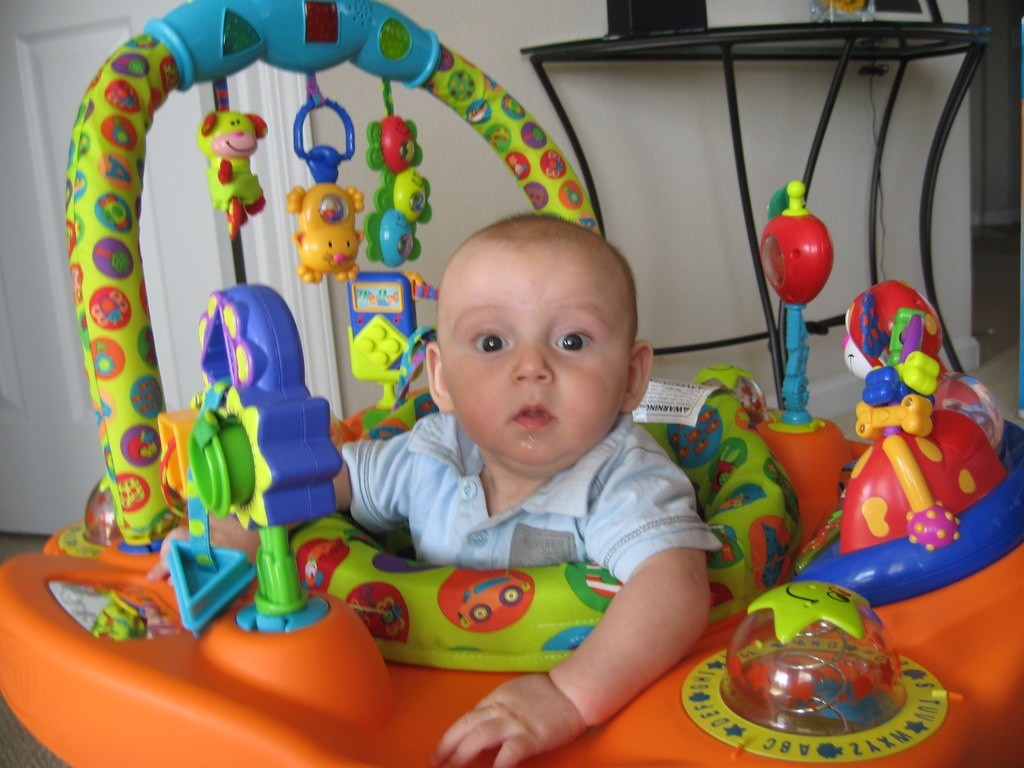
[862,0,942,22]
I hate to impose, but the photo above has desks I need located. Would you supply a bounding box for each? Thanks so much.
[520,21,992,412]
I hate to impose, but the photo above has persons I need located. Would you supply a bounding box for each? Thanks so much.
[149,213,722,768]
